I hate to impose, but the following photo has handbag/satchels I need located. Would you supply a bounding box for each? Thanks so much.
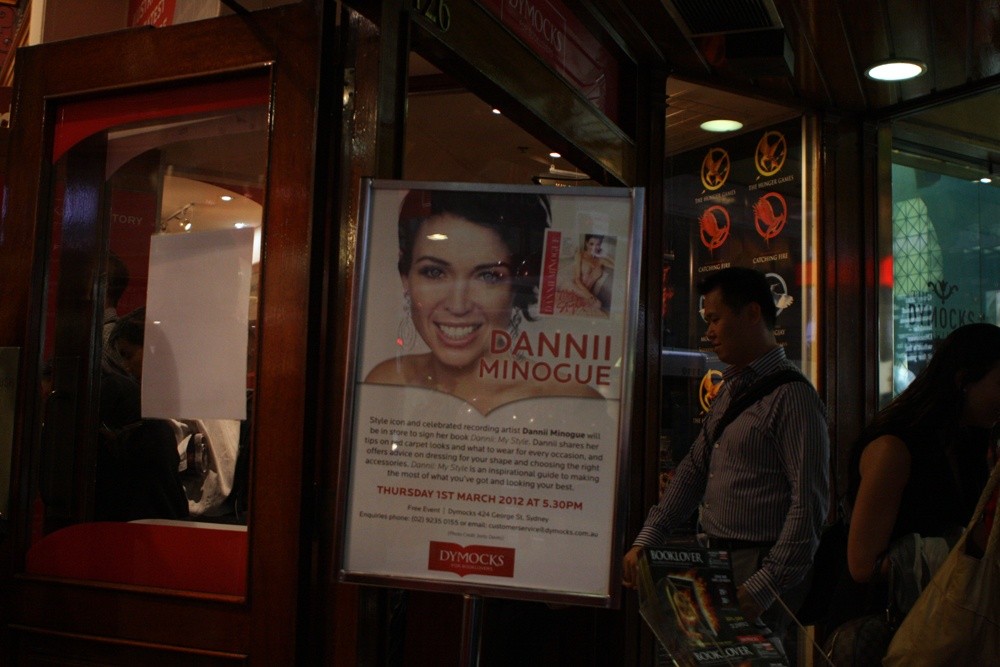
[790,519,889,627]
[880,462,1000,667]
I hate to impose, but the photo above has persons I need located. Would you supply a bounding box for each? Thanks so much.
[363,188,606,422]
[567,232,607,310]
[811,319,1000,667]
[95,254,199,521]
[616,264,834,619]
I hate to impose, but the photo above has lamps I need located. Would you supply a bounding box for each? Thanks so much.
[161,202,194,233]
